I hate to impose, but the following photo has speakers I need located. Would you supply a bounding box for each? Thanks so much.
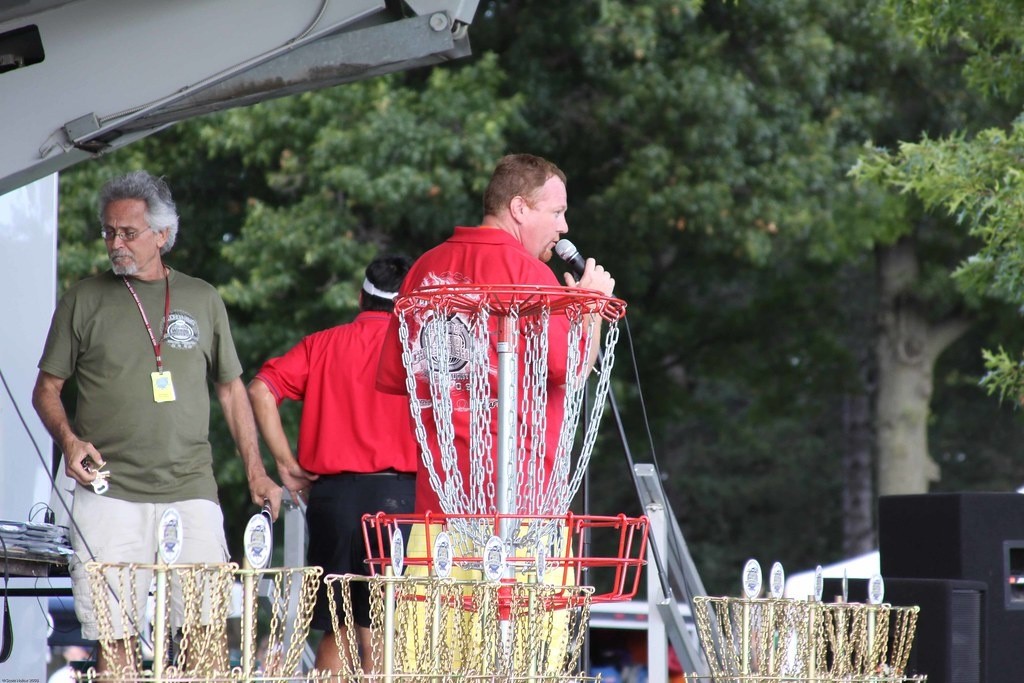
[821,577,989,683]
[878,491,1024,683]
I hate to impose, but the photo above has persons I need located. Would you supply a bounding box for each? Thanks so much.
[31,174,283,683]
[248,252,420,682]
[376,154,614,676]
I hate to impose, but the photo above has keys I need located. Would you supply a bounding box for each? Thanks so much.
[81,458,110,494]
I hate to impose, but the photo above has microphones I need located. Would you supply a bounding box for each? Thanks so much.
[554,237,620,298]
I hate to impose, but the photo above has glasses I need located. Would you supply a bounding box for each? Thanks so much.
[100,225,151,241]
